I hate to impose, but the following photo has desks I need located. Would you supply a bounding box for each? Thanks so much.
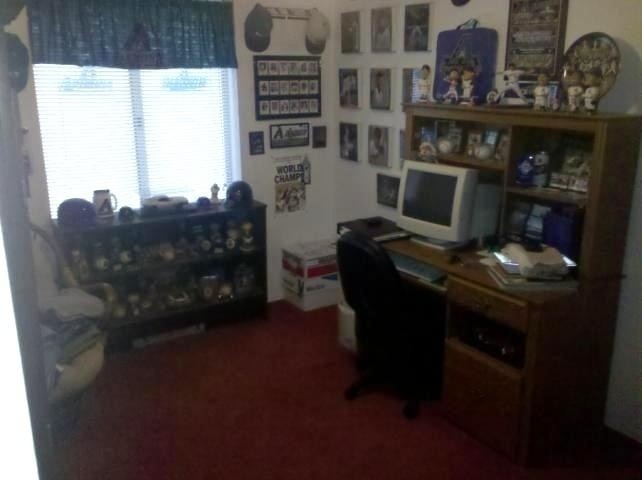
[340,220,581,472]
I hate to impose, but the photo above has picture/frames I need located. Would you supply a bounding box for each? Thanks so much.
[337,121,358,165]
[367,123,390,168]
[312,126,326,149]
[338,68,358,109]
[370,7,393,54]
[252,54,322,121]
[368,68,391,112]
[339,10,360,55]
[269,123,310,149]
[402,68,421,113]
[249,131,264,155]
[403,3,429,51]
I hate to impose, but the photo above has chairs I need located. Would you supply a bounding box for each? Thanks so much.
[336,230,442,420]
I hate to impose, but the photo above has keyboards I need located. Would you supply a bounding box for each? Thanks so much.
[390,255,445,282]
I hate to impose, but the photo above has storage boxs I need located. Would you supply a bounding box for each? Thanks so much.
[281,239,339,311]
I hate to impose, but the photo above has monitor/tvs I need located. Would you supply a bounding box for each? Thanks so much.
[395,159,500,250]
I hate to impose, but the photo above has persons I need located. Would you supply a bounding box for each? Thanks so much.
[582,67,603,113]
[532,73,550,113]
[437,68,458,102]
[567,70,585,114]
[512,155,534,185]
[490,61,534,110]
[462,66,485,104]
[375,15,390,49]
[532,151,551,187]
[372,72,384,107]
[417,142,438,162]
[70,217,257,283]
[418,64,431,104]
[370,129,384,164]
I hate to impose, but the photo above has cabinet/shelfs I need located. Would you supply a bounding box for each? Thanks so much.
[400,102,641,279]
[50,198,267,353]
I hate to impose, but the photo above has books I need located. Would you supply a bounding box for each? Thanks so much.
[337,216,412,244]
[486,250,581,293]
